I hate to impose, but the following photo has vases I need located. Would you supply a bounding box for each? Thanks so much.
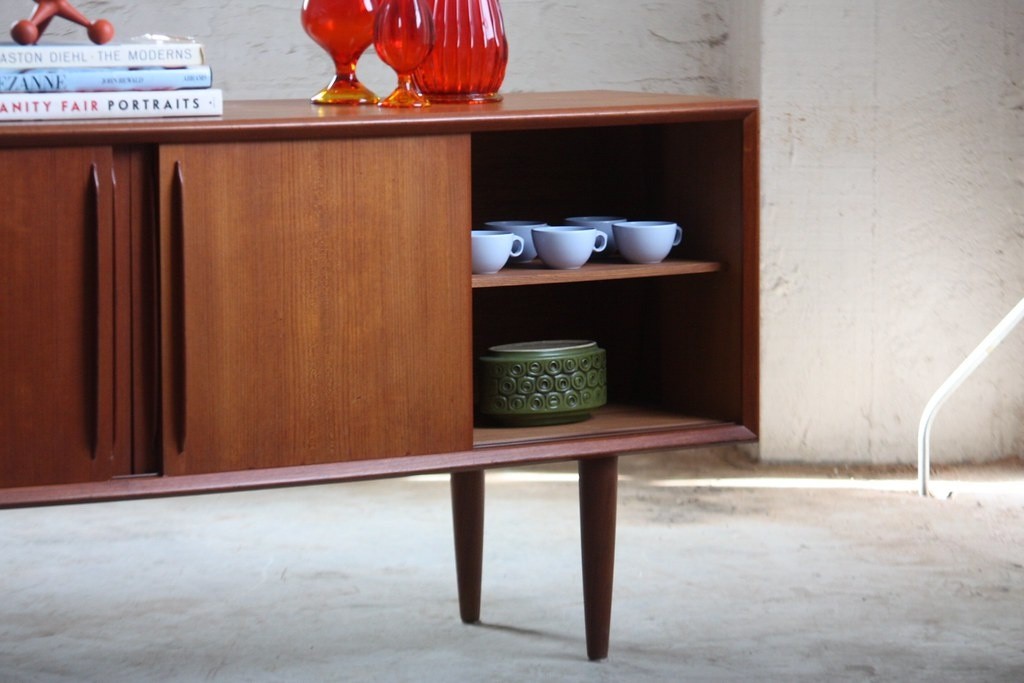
[372,0,436,110]
[300,0,382,105]
[413,0,509,105]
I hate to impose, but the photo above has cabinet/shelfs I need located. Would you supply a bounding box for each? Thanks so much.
[0,91,760,664]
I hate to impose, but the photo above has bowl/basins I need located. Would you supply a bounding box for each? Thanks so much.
[480,339,609,427]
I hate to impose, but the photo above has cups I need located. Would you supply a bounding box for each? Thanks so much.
[565,216,627,255]
[611,222,683,263]
[485,222,550,262]
[472,231,524,274]
[531,227,608,270]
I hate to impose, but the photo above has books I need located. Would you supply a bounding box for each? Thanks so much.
[0,33,223,120]
